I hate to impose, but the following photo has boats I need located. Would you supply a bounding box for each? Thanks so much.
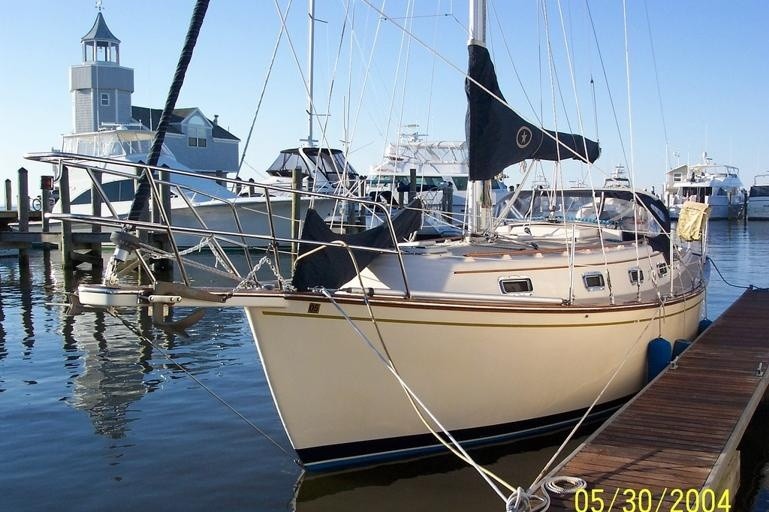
[9,0,716,481]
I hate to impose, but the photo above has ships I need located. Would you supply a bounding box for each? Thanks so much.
[576,153,768,227]
